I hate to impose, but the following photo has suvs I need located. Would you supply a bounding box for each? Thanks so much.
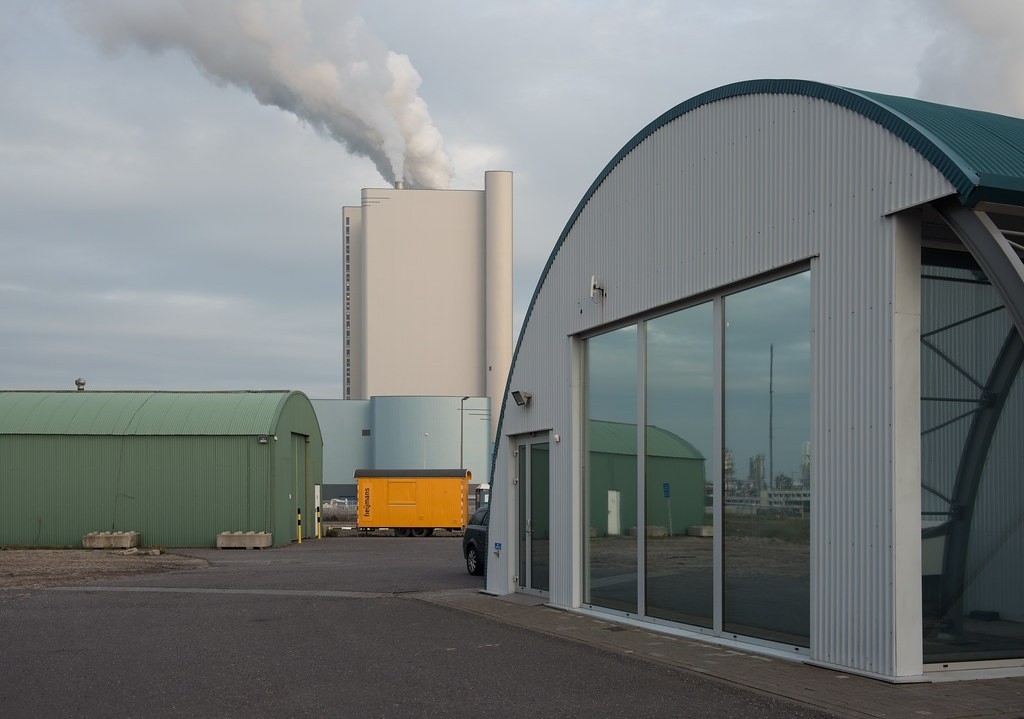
[463,505,489,575]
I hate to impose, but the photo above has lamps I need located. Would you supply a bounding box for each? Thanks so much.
[274,435,278,441]
[511,389,532,407]
[589,276,605,304]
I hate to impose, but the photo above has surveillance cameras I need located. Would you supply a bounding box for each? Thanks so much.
[272,435,278,443]
